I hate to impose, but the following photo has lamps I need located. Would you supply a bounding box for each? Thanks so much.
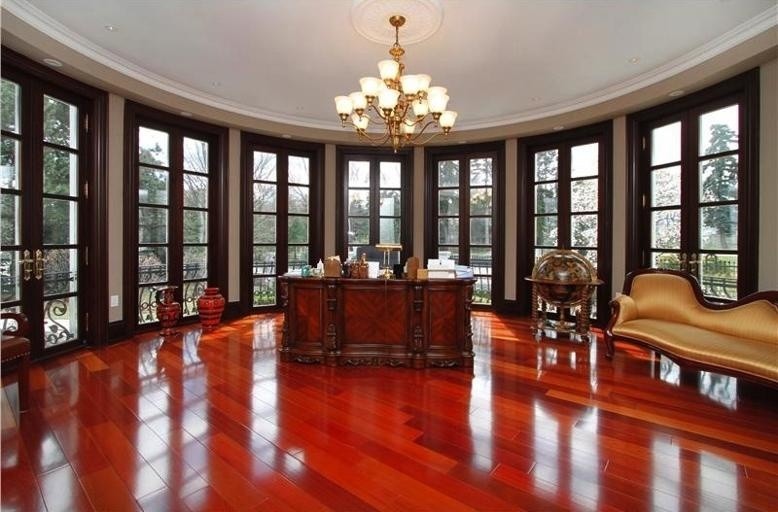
[374,243,404,280]
[333,14,459,154]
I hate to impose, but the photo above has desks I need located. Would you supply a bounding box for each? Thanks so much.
[274,271,478,371]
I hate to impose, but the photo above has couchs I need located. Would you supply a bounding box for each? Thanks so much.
[602,266,778,390]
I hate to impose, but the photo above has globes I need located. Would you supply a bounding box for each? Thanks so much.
[524,250,605,345]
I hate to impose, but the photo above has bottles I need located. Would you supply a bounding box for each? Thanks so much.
[299,253,369,279]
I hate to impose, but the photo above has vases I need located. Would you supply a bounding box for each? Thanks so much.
[196,287,227,332]
[155,285,182,338]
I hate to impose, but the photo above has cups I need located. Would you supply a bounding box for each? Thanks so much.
[385,256,428,280]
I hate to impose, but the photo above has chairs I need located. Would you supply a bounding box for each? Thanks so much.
[356,244,399,270]
[0,309,33,415]
